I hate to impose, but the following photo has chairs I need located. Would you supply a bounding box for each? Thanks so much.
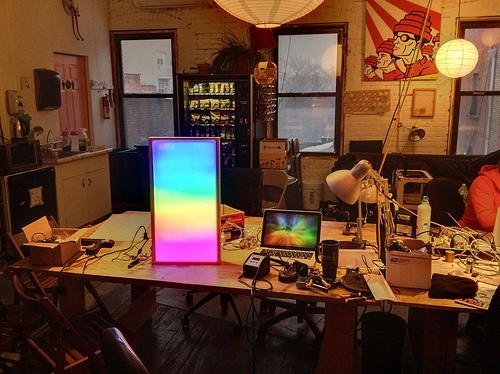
[262,169,291,214]
[7,216,151,374]
[180,168,263,334]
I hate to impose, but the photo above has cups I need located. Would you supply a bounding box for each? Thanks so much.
[314,240,340,282]
[445,250,455,262]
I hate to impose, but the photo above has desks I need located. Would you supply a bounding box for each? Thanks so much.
[6,211,500,374]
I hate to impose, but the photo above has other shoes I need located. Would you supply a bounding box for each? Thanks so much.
[464,324,484,338]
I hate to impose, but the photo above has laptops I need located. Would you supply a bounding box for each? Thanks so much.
[256,209,323,268]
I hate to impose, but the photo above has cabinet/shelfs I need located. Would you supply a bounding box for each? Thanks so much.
[44,152,112,228]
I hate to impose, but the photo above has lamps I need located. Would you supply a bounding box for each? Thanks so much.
[435,0,479,79]
[325,160,394,275]
[408,125,426,142]
[214,0,325,29]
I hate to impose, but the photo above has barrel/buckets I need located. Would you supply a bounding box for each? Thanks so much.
[301,180,324,210]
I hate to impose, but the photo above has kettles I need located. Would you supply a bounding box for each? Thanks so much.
[10,114,30,142]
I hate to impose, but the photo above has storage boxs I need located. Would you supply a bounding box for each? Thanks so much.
[259,138,289,170]
[220,204,244,244]
[385,237,433,289]
[22,216,90,265]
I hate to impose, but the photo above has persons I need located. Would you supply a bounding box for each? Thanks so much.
[456,148,500,337]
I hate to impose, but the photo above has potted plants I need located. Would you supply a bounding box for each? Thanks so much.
[208,29,267,75]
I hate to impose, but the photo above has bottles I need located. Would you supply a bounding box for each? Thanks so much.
[62,127,90,153]
[416,196,432,244]
[458,183,469,205]
